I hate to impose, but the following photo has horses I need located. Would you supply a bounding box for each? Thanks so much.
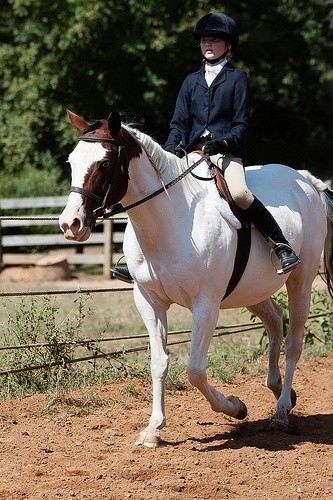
[58,108,333,449]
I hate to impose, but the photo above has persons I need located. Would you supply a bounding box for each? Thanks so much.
[111,12,299,282]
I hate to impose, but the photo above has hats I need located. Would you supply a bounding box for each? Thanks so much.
[193,13,237,41]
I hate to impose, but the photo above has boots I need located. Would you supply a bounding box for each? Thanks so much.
[109,265,133,282]
[242,195,298,272]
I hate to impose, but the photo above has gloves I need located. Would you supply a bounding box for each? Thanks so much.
[164,145,176,153]
[204,138,227,155]
